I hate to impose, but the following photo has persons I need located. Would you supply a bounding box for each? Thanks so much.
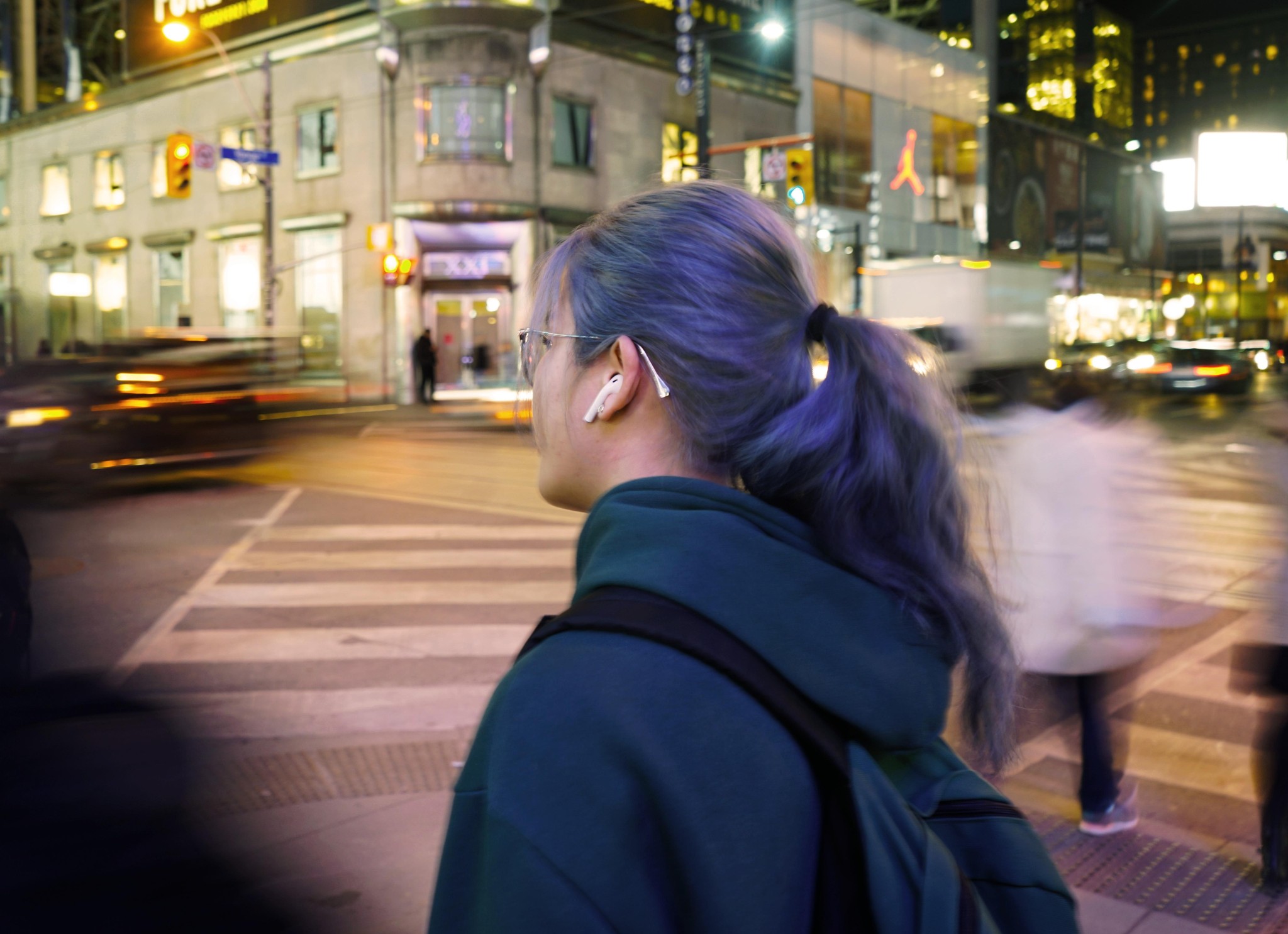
[426,179,1084,934]
[984,374,1194,834]
[412,329,440,404]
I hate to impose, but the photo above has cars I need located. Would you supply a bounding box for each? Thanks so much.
[1054,333,1288,400]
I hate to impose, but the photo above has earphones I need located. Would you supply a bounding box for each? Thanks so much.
[584,374,624,423]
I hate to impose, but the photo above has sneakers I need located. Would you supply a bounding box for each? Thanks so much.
[1080,804,1140,835]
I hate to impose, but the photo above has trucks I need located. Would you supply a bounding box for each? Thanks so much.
[857,255,1083,411]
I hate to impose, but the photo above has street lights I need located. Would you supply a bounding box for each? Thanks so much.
[162,19,274,365]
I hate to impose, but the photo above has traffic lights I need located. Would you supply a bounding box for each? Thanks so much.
[381,254,418,286]
[167,127,192,199]
[783,150,814,209]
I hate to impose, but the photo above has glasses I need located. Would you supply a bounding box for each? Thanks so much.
[517,330,670,399]
